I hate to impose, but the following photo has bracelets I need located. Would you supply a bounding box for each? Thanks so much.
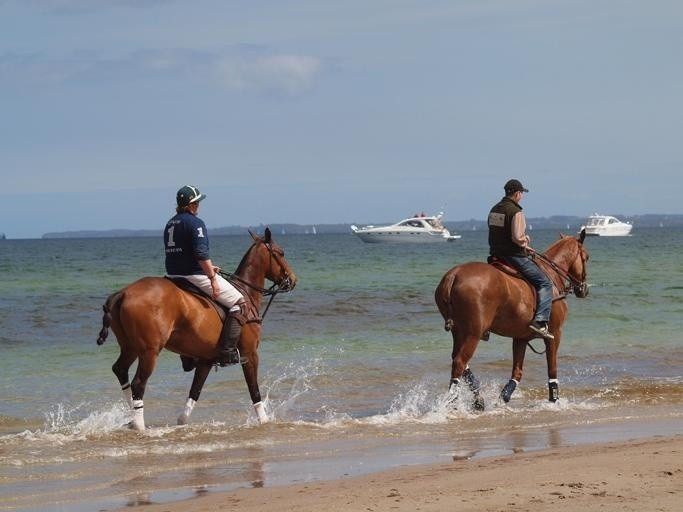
[208,274,216,279]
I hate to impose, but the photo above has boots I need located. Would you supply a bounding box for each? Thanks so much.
[180,355,198,372]
[220,310,249,368]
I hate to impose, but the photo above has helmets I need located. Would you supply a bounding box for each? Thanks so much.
[176,186,206,209]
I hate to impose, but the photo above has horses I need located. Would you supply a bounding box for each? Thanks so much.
[96,227,298,433]
[434,228,590,415]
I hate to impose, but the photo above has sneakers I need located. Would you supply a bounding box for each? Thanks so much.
[529,321,556,340]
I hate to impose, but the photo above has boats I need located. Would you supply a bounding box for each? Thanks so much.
[349,211,462,242]
[576,214,633,237]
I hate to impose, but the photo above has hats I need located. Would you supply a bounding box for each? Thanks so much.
[504,180,529,192]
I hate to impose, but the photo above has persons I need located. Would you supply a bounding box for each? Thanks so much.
[488,179,555,341]
[164,184,249,372]
